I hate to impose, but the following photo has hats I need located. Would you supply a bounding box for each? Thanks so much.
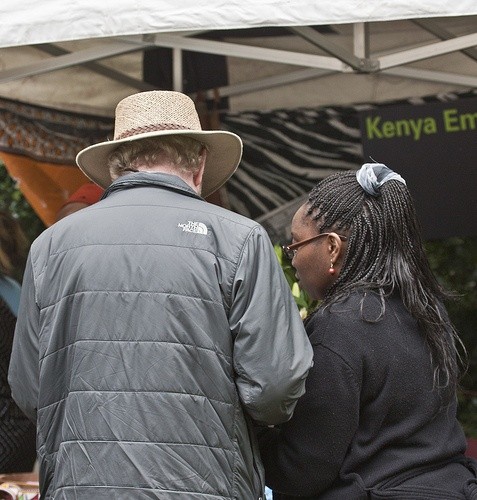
[75,90,244,198]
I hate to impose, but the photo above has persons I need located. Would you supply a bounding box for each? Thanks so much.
[8,90,314,500]
[257,163,477,500]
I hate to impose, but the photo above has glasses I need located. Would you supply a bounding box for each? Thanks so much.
[279,233,329,259]
